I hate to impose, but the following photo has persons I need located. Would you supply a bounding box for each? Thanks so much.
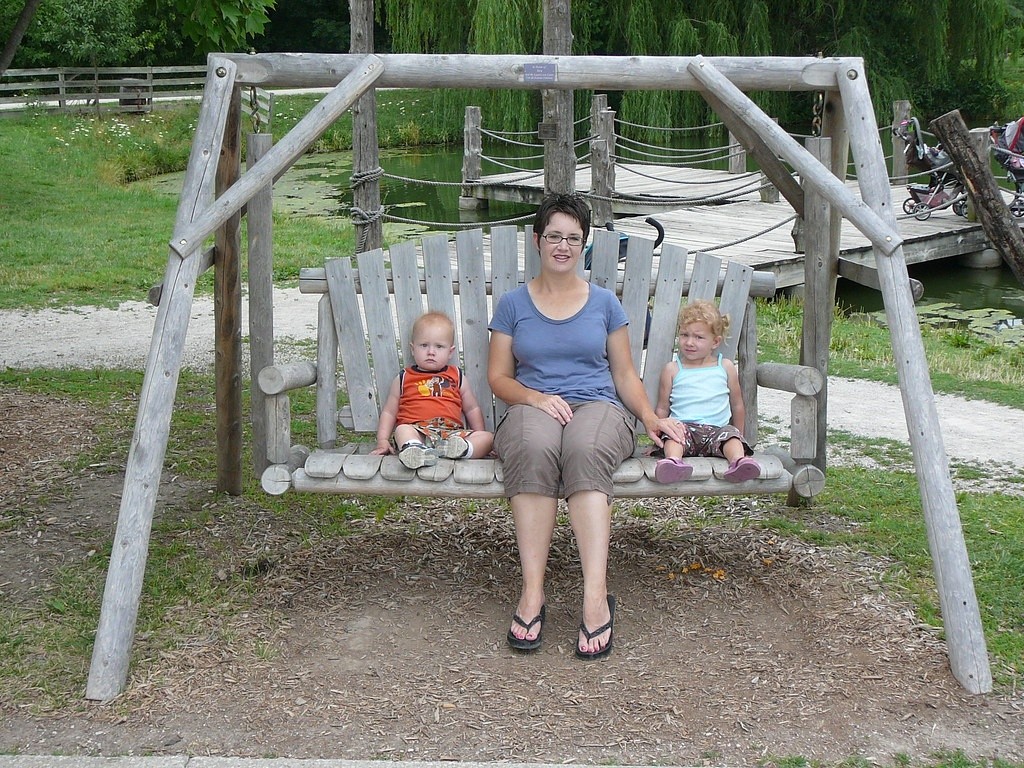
[651,300,762,484]
[370,310,494,470]
[487,196,684,659]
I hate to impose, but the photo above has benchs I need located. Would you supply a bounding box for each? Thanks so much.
[258,223,825,497]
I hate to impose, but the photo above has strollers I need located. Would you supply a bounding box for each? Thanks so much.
[988,117,1024,217]
[892,117,969,222]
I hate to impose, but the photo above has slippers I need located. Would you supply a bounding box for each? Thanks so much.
[724,455,763,484]
[574,593,617,660]
[506,606,545,650]
[655,458,692,483]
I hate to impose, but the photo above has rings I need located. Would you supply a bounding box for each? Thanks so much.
[675,422,680,426]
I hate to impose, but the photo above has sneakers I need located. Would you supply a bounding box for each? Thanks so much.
[436,436,468,460]
[396,443,437,469]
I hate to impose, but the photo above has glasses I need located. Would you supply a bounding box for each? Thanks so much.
[538,232,584,247]
